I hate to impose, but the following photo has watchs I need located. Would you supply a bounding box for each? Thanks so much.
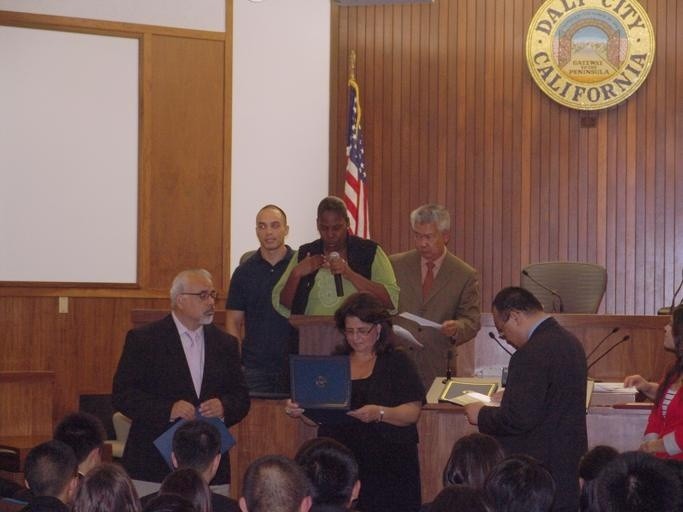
[378,406,384,423]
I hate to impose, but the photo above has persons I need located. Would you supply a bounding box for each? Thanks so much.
[271,196,402,321]
[385,203,480,392]
[1,412,143,512]
[240,436,360,512]
[424,432,556,511]
[283,291,423,510]
[225,204,299,400]
[577,445,683,512]
[112,268,250,499]
[461,286,587,508]
[639,299,682,465]
[140,419,244,512]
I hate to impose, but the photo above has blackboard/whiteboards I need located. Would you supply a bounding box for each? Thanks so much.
[0,24,142,287]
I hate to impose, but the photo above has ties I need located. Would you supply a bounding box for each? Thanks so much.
[423,261,435,303]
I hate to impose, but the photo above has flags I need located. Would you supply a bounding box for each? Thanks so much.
[335,50,370,243]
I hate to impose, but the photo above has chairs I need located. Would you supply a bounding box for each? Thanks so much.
[518,261,609,313]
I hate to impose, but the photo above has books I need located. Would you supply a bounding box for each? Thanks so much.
[589,382,638,406]
[153,402,238,472]
[287,353,365,426]
[438,379,498,404]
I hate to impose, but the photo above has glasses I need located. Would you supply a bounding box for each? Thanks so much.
[344,324,375,336]
[181,290,217,301]
[497,311,512,339]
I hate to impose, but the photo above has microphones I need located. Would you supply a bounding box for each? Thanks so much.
[488,332,511,355]
[522,270,563,313]
[329,251,344,297]
[442,349,453,383]
[587,326,620,361]
[587,335,629,370]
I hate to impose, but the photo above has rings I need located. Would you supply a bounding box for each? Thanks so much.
[283,406,291,416]
